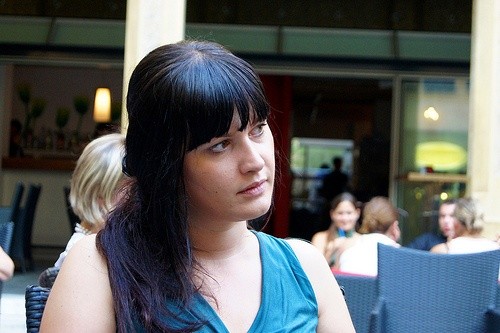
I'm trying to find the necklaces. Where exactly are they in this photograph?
[74,222,94,235]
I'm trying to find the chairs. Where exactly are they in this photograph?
[333,242,500,333]
[1,182,42,298]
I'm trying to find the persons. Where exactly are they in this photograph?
[337,196,402,277]
[38,38,357,333]
[310,192,361,268]
[50,134,136,269]
[0,244,15,282]
[427,196,500,255]
[405,198,460,252]
[311,157,352,229]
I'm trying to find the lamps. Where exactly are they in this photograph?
[93,88,111,123]
